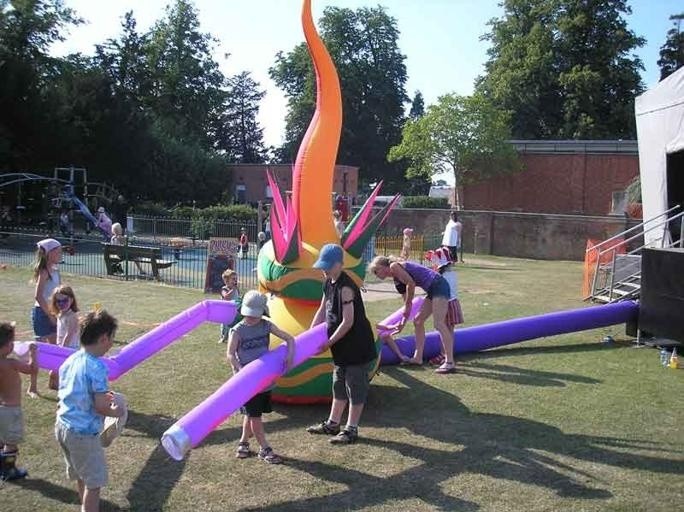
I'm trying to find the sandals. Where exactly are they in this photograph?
[400,353,456,374]
[258,447,283,463]
[235,442,249,458]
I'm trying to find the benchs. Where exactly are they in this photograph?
[104,243,178,277]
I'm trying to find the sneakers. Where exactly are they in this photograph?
[306,422,358,444]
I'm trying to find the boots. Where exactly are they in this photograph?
[0,447,28,481]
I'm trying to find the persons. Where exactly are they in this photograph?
[258,206,271,249]
[96,207,112,235]
[234,175,247,203]
[54,310,124,512]
[220,268,241,342]
[335,194,357,222]
[399,228,413,262]
[441,211,462,265]
[60,210,68,224]
[368,246,464,373]
[48,285,80,390]
[264,181,272,198]
[0,204,17,229]
[0,321,27,481]
[305,243,379,443]
[26,238,62,399]
[111,222,146,274]
[240,227,249,259]
[227,290,294,463]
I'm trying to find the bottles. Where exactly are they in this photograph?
[660,345,668,364]
[670,348,680,369]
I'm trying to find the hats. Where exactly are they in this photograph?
[240,290,269,317]
[98,206,104,212]
[425,246,453,270]
[100,391,129,447]
[312,244,343,269]
